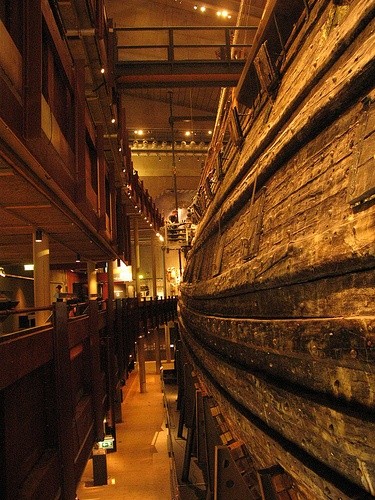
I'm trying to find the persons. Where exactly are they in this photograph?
[53,285,63,302]
[169,210,178,242]
